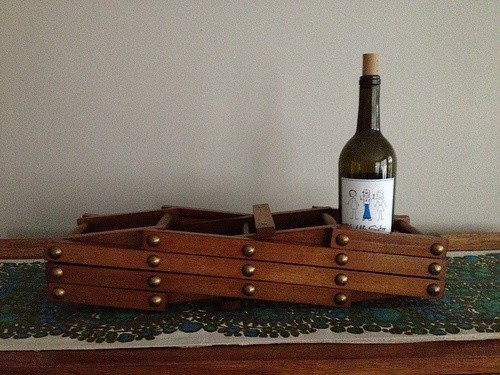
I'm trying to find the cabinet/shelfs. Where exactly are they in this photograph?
[45,203,449,311]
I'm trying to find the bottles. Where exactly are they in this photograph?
[339,52,398,234]
